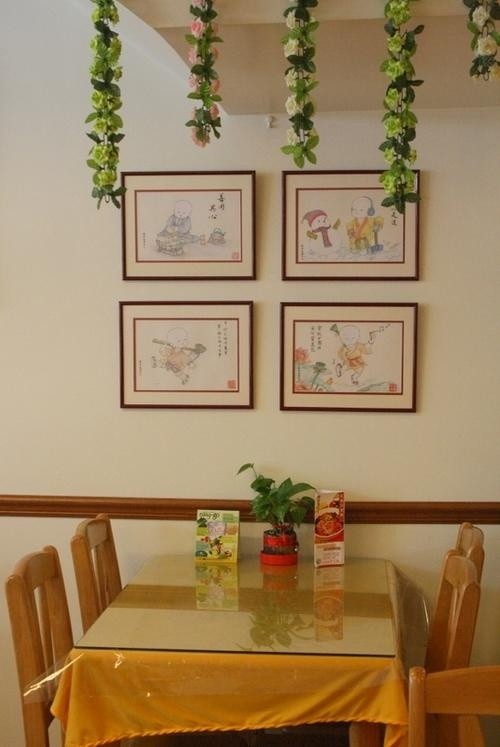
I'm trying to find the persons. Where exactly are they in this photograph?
[166,327,196,386]
[346,197,384,257]
[335,325,375,385]
[156,200,200,244]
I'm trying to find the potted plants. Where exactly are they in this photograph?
[236,462,318,565]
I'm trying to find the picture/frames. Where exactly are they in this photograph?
[282,167,420,282]
[119,299,254,410]
[120,171,258,281]
[279,301,418,412]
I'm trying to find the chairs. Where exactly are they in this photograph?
[455,523,484,583]
[424,549,482,672]
[6,545,75,745]
[407,665,500,746]
[70,513,123,634]
